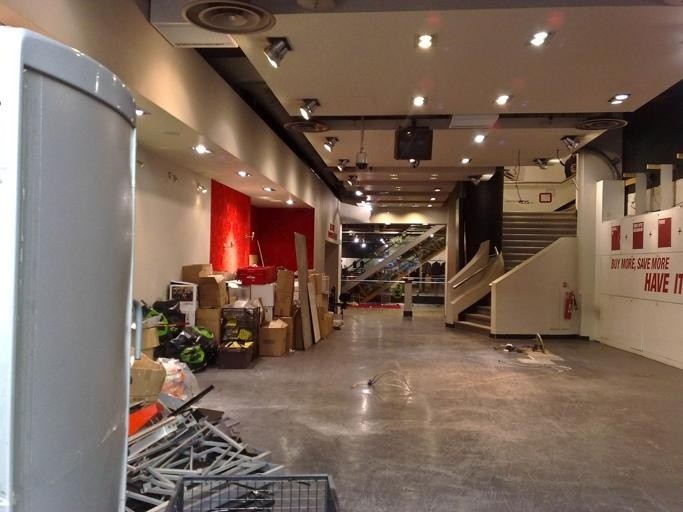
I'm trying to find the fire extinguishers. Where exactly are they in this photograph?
[565,290,579,320]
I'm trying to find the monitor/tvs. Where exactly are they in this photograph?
[395,129,433,160]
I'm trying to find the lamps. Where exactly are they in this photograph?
[262,37,357,188]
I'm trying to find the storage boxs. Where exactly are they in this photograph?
[165,264,335,367]
